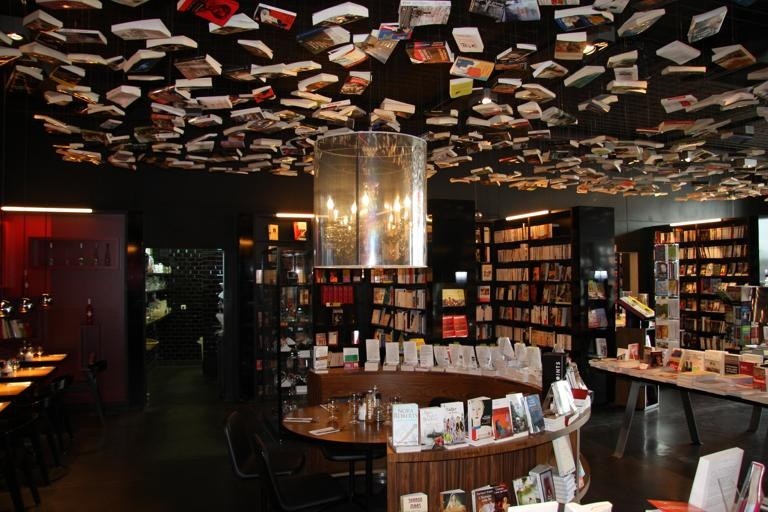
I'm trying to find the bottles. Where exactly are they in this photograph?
[348,383,400,426]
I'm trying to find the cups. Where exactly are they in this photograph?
[326,399,341,431]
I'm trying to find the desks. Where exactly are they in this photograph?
[281,398,406,512]
[1,352,67,511]
[586,352,766,459]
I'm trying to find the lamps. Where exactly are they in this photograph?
[311,131,431,273]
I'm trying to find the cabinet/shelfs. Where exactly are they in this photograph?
[145,272,173,326]
[252,202,767,440]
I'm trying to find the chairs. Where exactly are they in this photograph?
[222,410,346,512]
[321,393,387,497]
[58,357,108,440]
